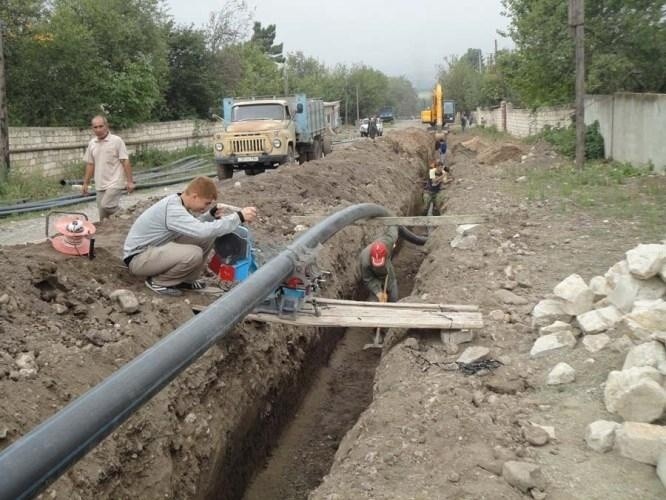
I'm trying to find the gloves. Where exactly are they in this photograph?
[376,292,389,302]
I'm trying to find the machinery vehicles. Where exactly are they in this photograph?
[419,83,464,144]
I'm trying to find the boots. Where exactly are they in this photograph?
[423,208,428,216]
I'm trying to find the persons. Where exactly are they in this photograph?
[438,137,447,164]
[121,175,258,296]
[353,237,399,337]
[81,114,132,222]
[461,112,467,133]
[420,159,453,213]
[441,166,450,181]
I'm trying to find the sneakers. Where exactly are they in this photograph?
[145,277,184,297]
[168,279,204,289]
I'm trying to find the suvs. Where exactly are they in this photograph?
[358,117,383,137]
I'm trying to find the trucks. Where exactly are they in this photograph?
[378,105,394,122]
[211,93,334,181]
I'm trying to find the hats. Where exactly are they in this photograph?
[371,240,388,268]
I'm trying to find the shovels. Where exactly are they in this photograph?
[362,265,389,351]
